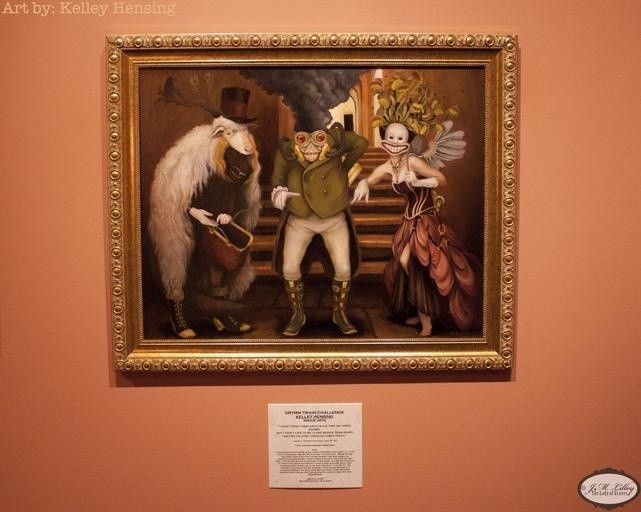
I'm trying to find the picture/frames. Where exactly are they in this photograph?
[105,32,516,375]
